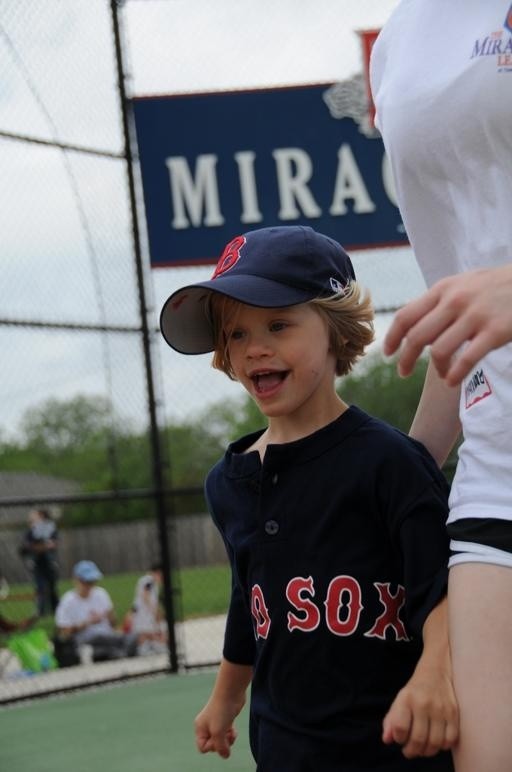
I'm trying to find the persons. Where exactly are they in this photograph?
[0,560,169,687]
[161,225,460,772]
[365,0,512,772]
[34,509,62,569]
[18,507,64,617]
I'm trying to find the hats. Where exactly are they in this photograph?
[160,224,356,355]
[73,560,104,582]
